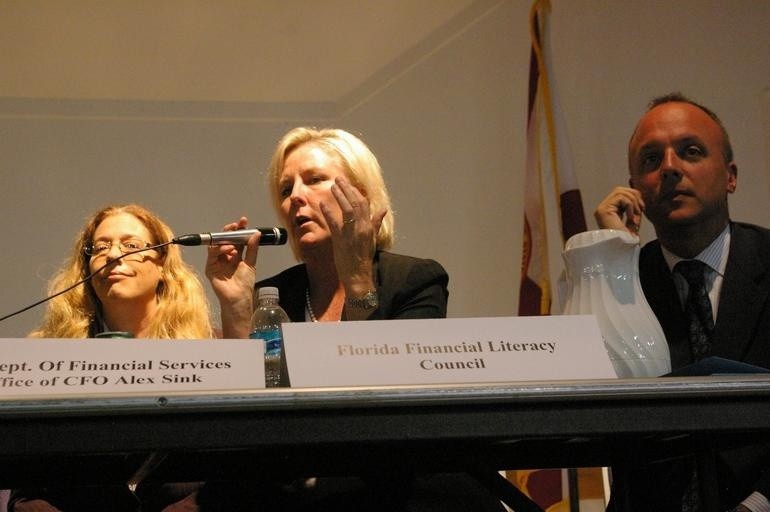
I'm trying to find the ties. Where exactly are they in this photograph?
[677,261,717,360]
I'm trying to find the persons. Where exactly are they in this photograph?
[204,125,506,511]
[593,91,770,511]
[6,203,202,512]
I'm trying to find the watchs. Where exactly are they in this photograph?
[339,292,383,312]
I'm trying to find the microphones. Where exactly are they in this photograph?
[172,225,290,250]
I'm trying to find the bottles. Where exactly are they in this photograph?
[247,285,292,390]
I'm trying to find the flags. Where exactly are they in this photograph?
[515,14,588,315]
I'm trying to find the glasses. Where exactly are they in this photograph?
[82,234,159,259]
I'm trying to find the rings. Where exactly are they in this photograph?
[342,216,357,223]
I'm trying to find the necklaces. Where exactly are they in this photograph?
[306,286,344,323]
